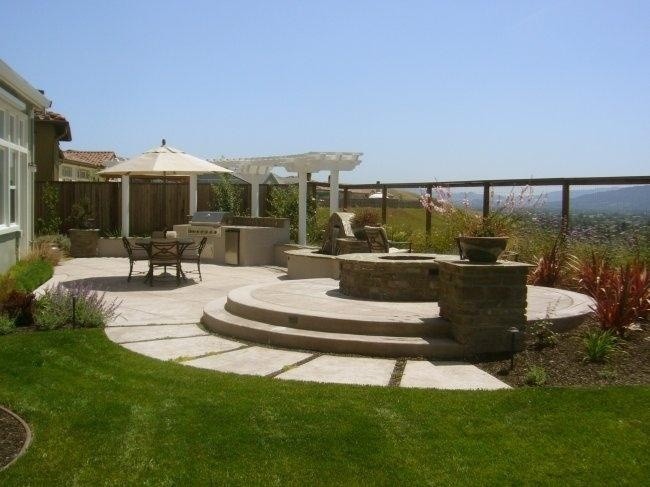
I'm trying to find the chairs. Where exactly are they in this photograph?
[122,235,207,287]
[364,225,412,252]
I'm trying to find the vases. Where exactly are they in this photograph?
[458,237,508,264]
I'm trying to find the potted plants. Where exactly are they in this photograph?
[66,194,102,257]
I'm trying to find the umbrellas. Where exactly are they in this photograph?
[95,137,234,229]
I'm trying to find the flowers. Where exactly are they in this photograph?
[419,176,547,237]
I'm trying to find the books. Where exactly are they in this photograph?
[166,224,191,239]
